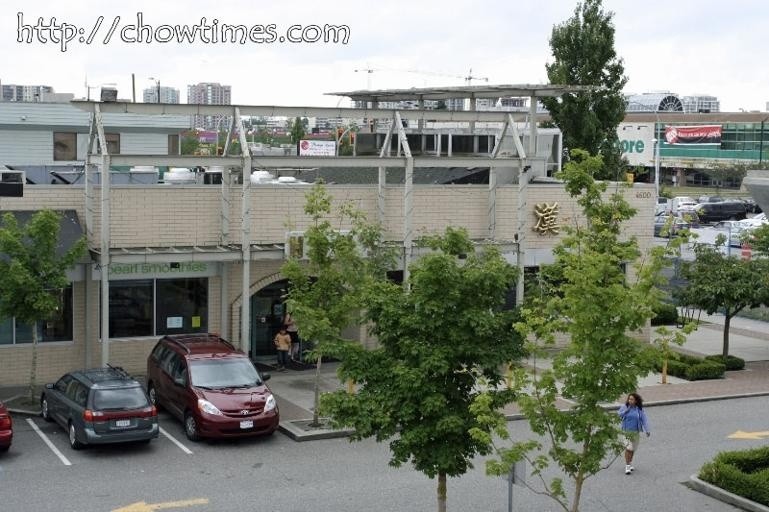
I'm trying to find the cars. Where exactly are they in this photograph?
[654,194,769,247]
[42,367,159,450]
[0,400,13,451]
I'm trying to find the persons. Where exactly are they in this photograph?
[617,392,651,474]
[285,312,301,362]
[272,328,291,372]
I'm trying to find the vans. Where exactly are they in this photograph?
[148,334,279,442]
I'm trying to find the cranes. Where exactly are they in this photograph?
[354,65,488,90]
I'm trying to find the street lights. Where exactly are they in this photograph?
[148,78,160,103]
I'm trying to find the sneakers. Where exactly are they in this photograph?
[624,462,635,474]
